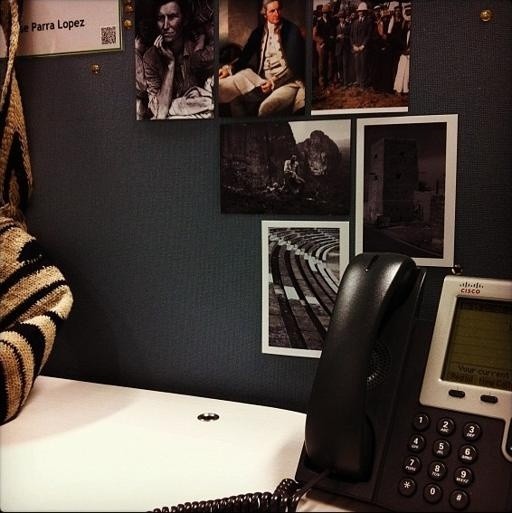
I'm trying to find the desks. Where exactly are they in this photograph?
[1,374,308,513]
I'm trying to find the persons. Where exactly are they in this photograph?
[284,154,300,195]
[142,1,215,121]
[312,0,411,96]
[218,0,305,117]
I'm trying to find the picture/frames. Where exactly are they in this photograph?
[354,114,458,271]
[260,221,349,358]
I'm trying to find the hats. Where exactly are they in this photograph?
[357,3,367,11]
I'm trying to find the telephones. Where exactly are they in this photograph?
[294,253,512,512]
